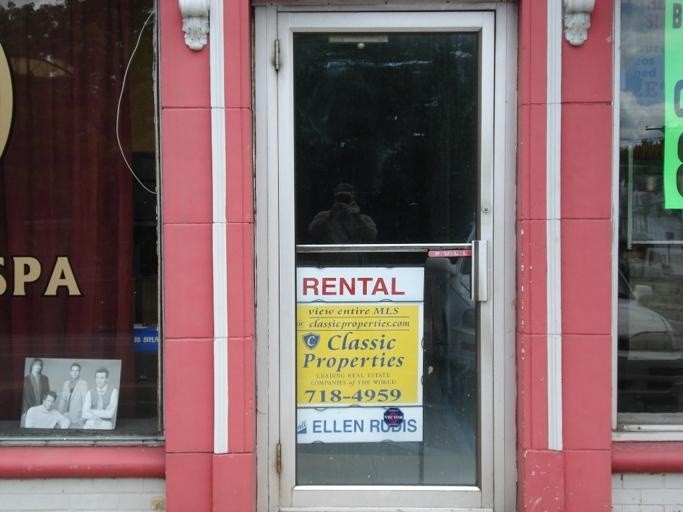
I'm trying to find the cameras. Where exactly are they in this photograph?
[333,191,352,205]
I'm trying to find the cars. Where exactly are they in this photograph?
[425,221,680,413]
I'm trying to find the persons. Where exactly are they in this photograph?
[20,359,117,430]
[307,181,378,267]
